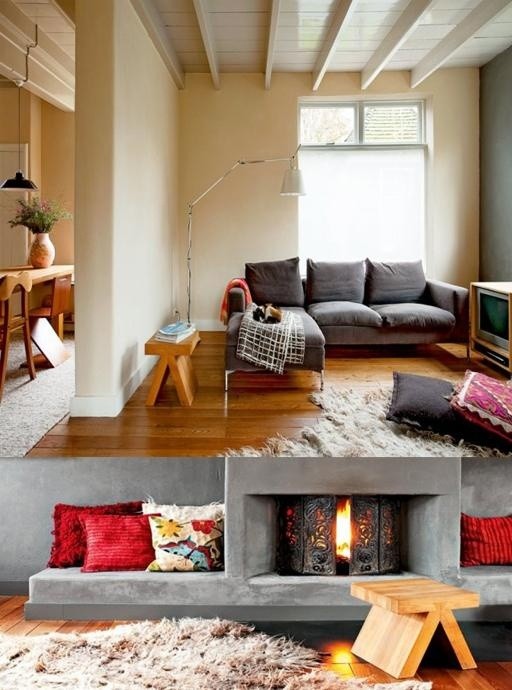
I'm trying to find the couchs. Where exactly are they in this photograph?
[224,274,470,390]
[30,458,511,626]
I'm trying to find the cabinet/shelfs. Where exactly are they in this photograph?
[469,281,512,375]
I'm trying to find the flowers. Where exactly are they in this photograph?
[6,195,72,232]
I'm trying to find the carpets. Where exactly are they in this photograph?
[217,379,512,457]
[0,327,77,457]
[1,616,434,690]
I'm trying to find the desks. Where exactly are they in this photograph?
[0,264,74,295]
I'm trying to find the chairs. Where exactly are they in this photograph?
[0,273,38,406]
[20,274,73,369]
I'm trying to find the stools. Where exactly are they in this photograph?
[145,330,201,407]
[351,578,480,679]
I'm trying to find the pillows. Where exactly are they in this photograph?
[48,501,151,568]
[145,516,225,572]
[141,502,225,522]
[79,513,160,573]
[461,512,512,568]
[451,369,512,442]
[387,372,457,437]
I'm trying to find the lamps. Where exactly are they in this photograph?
[0,78,39,191]
[185,144,306,325]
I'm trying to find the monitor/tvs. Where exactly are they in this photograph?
[476,289,510,348]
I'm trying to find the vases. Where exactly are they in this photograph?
[30,233,55,268]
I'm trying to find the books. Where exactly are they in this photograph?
[155,322,196,344]
